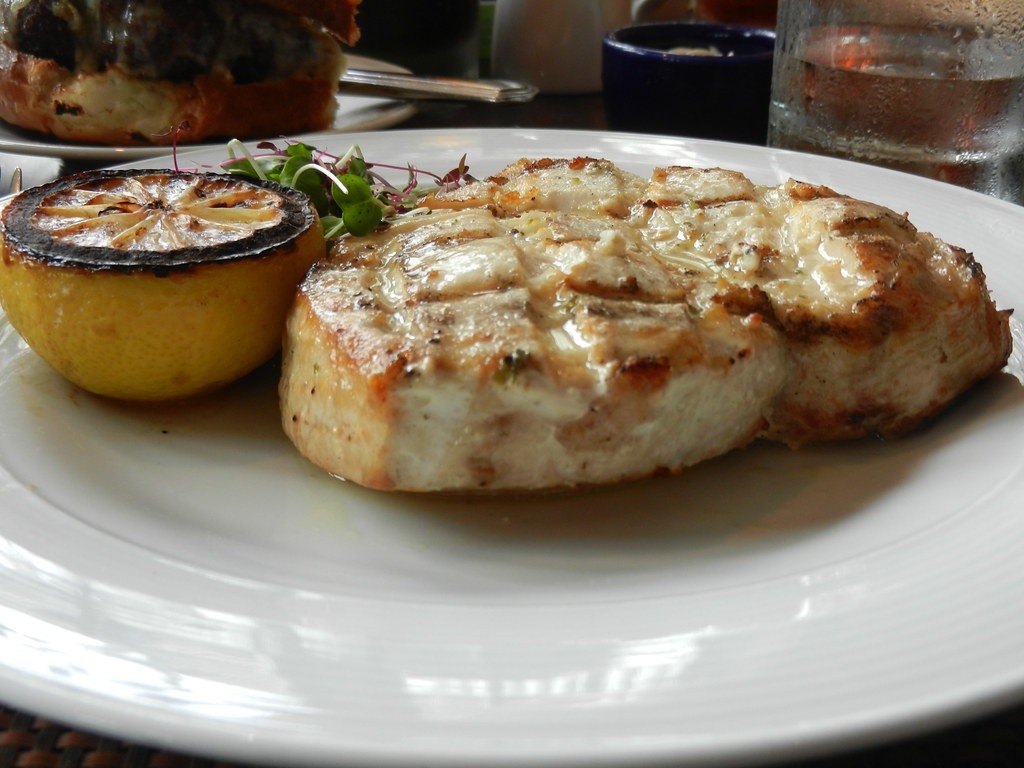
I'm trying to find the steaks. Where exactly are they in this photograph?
[280,150,1013,496]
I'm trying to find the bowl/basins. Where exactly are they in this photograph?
[603,23,776,160]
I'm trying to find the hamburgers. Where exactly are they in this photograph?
[0,0,358,140]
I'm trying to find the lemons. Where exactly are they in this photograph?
[1,168,321,401]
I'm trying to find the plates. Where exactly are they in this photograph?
[0,50,422,164]
[0,126,1024,766]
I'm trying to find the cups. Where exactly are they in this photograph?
[765,2,1022,219]
